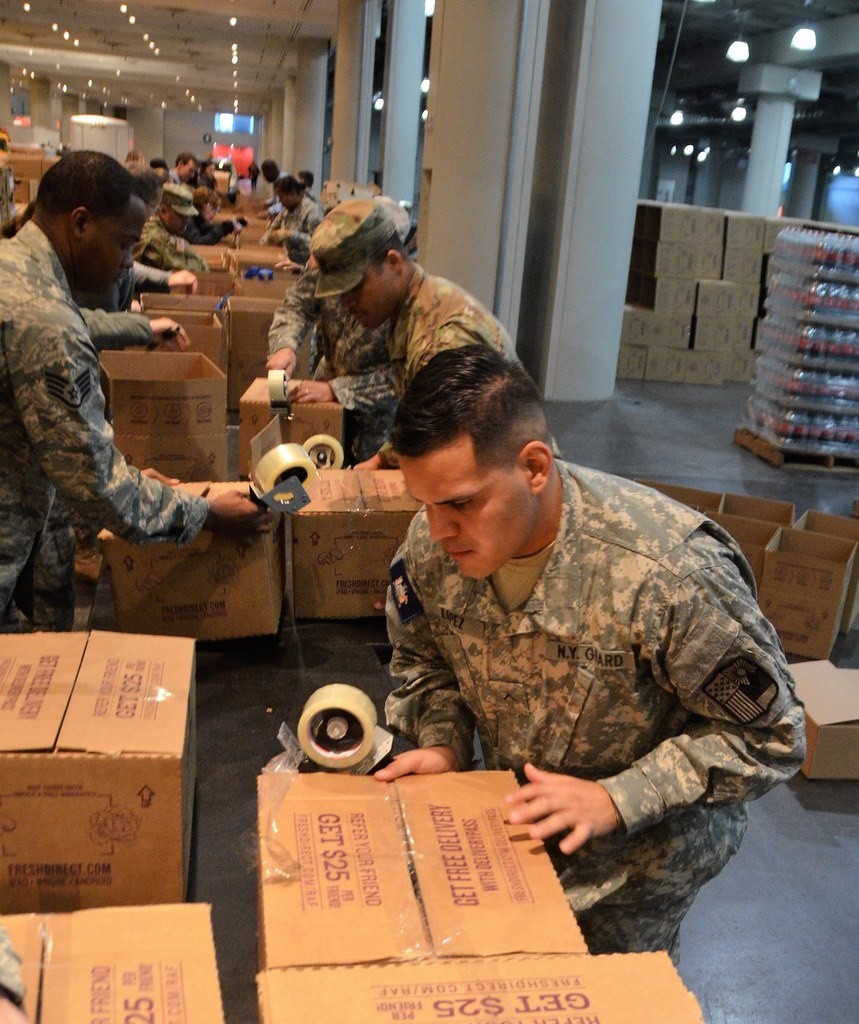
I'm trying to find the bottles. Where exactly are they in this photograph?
[742,226,859,455]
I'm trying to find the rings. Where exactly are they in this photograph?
[305,387,310,394]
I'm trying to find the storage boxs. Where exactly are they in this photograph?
[322,180,384,209]
[787,659,859,782]
[0,631,196,915]
[0,900,227,1024]
[169,171,300,298]
[7,144,63,231]
[239,376,344,477]
[97,481,285,641]
[139,292,311,409]
[287,468,425,620]
[255,767,588,970]
[634,477,859,662]
[254,950,706,1024]
[617,200,859,386]
[98,349,229,484]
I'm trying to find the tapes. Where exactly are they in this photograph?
[255,442,316,500]
[297,684,378,769]
[265,368,289,399]
[303,433,345,472]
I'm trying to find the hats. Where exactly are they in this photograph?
[161,184,200,216]
[372,195,412,244]
[310,199,395,298]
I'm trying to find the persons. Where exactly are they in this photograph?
[0,149,418,314]
[0,150,274,635]
[265,195,563,471]
[374,343,806,966]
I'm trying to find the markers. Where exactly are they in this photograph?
[145,325,178,352]
